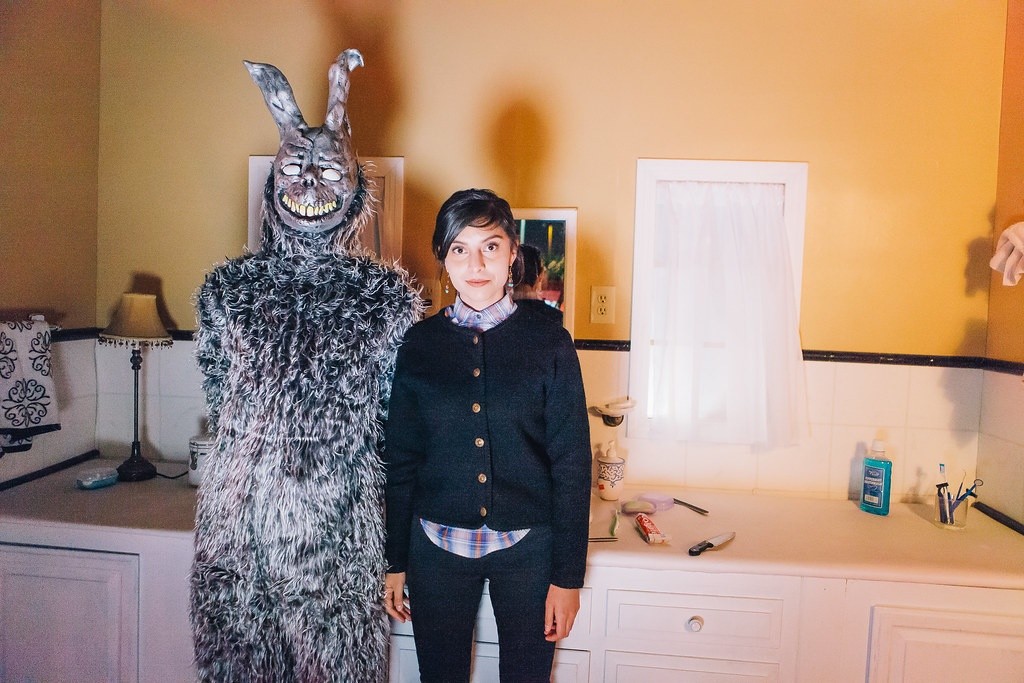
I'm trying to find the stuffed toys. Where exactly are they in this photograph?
[189,45,432,682]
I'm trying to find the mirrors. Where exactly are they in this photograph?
[508,207,578,344]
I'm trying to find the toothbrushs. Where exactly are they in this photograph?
[938,462,953,525]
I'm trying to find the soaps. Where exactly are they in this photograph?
[621,500,656,514]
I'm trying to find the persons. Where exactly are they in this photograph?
[510,244,564,327]
[379,182,592,683]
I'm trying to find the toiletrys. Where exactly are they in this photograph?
[859,438,893,516]
[633,512,673,545]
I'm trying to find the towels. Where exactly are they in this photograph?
[0,319,63,460]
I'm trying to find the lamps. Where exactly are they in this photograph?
[97,291,175,476]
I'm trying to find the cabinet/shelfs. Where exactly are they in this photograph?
[0,456,1023,682]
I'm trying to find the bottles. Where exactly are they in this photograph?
[858,439,894,518]
[186,435,217,489]
